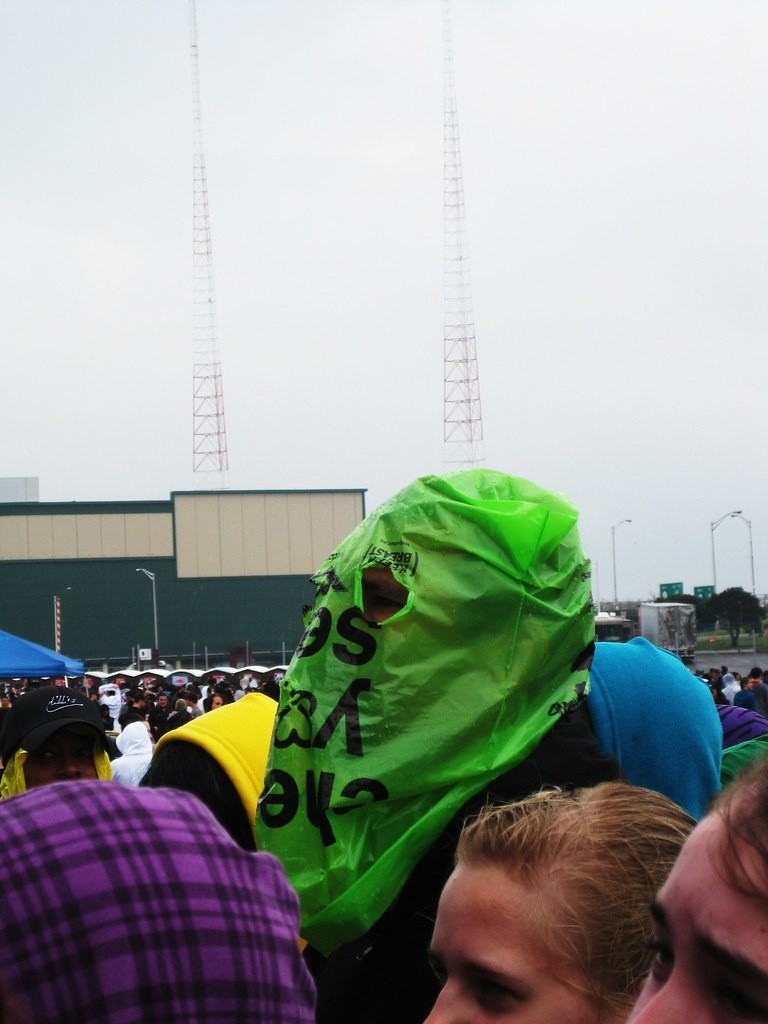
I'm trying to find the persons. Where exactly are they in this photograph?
[1,466,767,1023]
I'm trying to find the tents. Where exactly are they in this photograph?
[0,629,92,698]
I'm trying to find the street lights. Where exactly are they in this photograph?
[731,514,756,597]
[54,587,72,652]
[711,510,742,593]
[136,568,157,649]
[613,518,632,604]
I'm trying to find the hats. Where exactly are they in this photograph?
[3,685,111,765]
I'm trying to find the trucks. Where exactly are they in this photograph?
[638,601,698,664]
